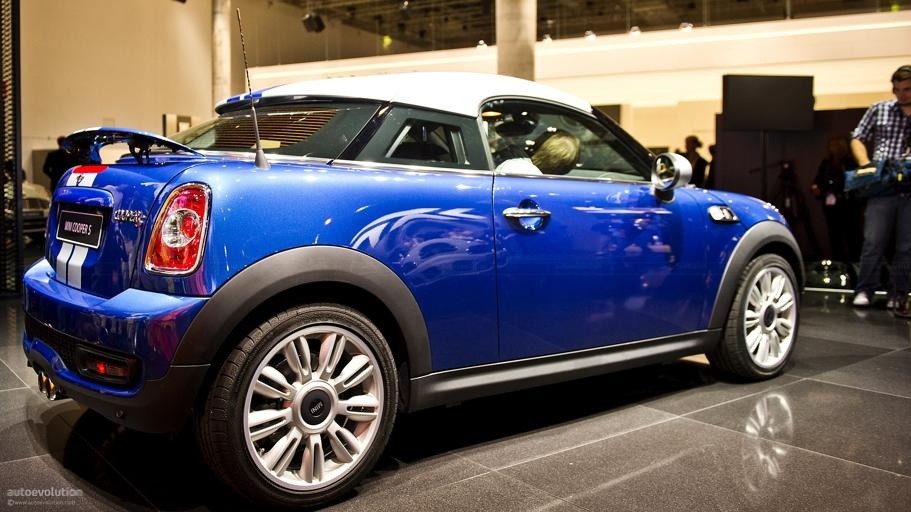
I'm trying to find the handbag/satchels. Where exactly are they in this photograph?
[844,157,910,200]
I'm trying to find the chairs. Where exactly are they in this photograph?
[397,141,447,161]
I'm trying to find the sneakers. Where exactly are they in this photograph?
[887,297,908,308]
[853,292,870,306]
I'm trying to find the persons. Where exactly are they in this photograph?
[494,128,582,176]
[42,136,72,195]
[810,137,858,262]
[679,135,707,185]
[848,65,911,309]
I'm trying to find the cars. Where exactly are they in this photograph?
[2,163,51,256]
[18,74,803,502]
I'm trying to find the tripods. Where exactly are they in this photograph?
[748,161,821,260]
